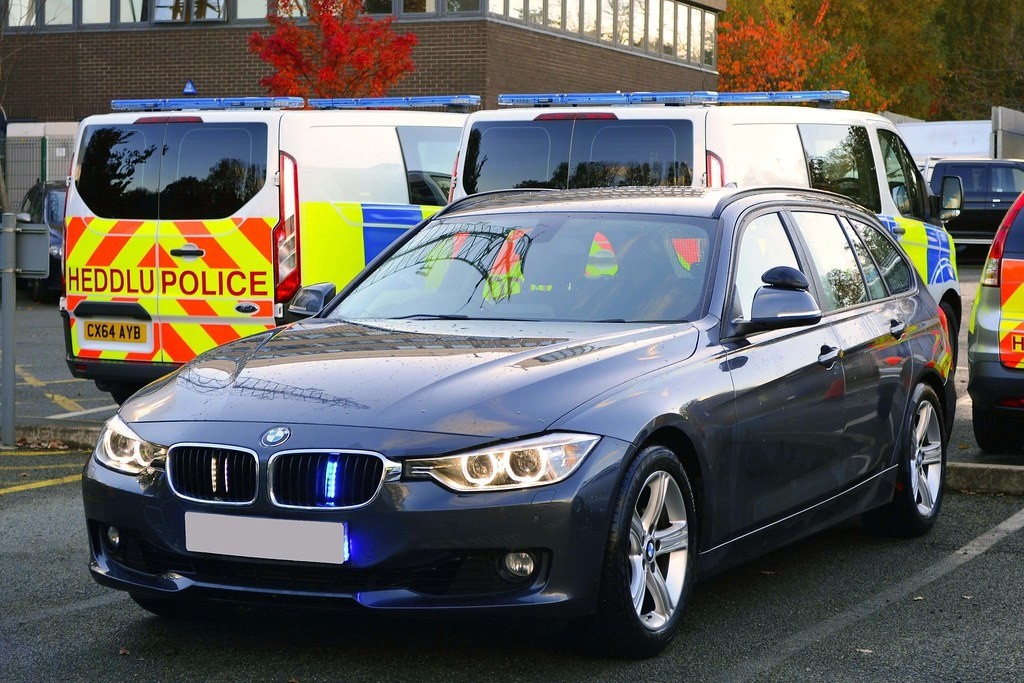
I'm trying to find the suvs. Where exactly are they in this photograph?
[14,177,68,297]
[900,156,1023,263]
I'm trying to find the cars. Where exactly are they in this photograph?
[965,186,1024,457]
[81,183,958,654]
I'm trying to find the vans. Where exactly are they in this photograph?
[60,86,965,401]
